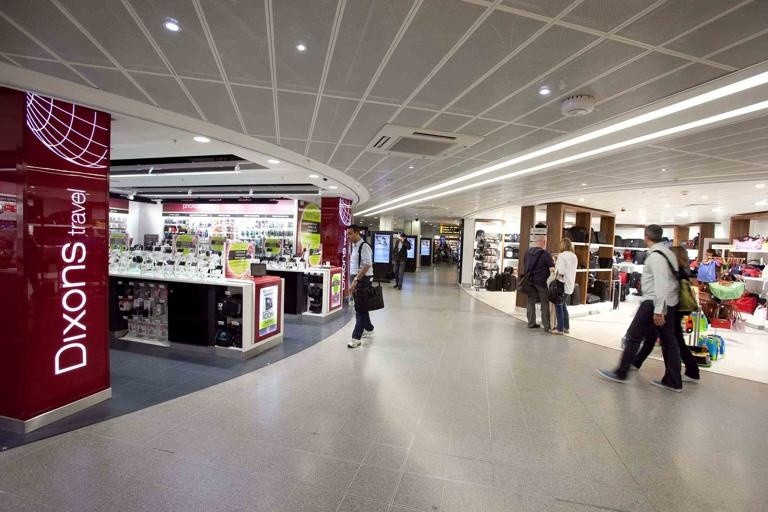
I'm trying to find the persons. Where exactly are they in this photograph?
[347,224,384,348]
[517,235,555,332]
[394,233,411,290]
[547,238,579,334]
[595,225,684,392]
[704,248,722,259]
[630,247,703,383]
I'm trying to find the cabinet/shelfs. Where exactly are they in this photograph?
[164,217,187,245]
[108,267,284,362]
[435,239,458,263]
[729,211,768,333]
[210,217,238,245]
[473,230,502,286]
[615,224,674,267]
[187,217,210,245]
[266,266,343,318]
[502,233,520,280]
[262,217,295,256]
[561,204,615,304]
[238,217,262,256]
[108,209,128,242]
[676,222,714,278]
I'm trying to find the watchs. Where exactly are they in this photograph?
[354,276,359,280]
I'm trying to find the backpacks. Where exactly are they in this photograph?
[353,242,384,312]
[548,270,565,306]
[517,249,547,294]
[504,245,514,258]
[652,250,699,313]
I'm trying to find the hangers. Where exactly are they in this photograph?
[698,257,745,324]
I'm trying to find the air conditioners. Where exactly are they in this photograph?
[365,124,486,161]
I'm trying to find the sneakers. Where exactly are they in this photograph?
[594,365,631,386]
[651,377,684,393]
[362,329,374,337]
[563,330,570,335]
[346,338,362,348]
[549,329,565,336]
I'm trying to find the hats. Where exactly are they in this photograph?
[400,231,406,237]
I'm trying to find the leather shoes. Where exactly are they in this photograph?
[393,283,397,289]
[528,324,542,328]
[398,287,402,290]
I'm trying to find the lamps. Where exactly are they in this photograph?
[128,191,136,199]
[235,163,240,171]
[560,95,595,117]
[682,191,689,195]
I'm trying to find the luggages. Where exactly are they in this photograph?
[503,273,516,292]
[485,273,496,290]
[495,271,504,292]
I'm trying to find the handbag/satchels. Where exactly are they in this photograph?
[598,257,614,268]
[732,231,768,251]
[697,262,716,284]
